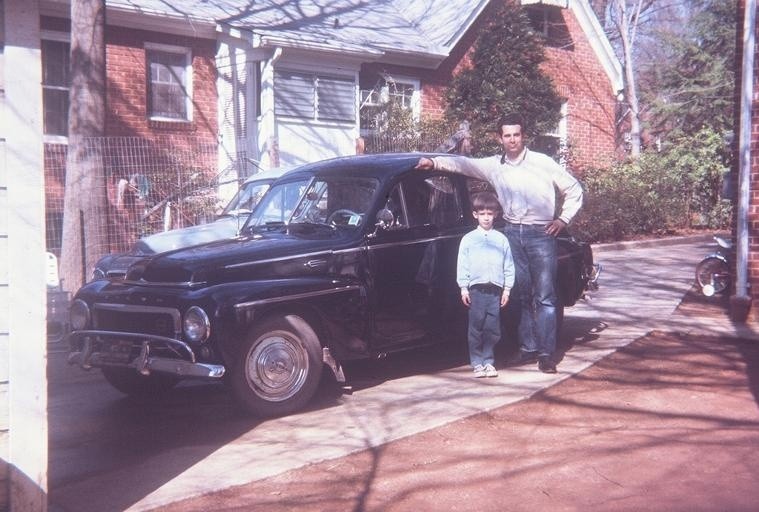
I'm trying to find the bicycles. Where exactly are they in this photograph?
[692,230,743,295]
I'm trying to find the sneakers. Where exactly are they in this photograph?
[485,363,498,377]
[473,365,486,378]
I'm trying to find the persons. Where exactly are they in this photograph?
[455,193,516,379]
[416,114,585,373]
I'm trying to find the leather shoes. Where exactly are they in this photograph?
[538,357,556,373]
[502,350,537,367]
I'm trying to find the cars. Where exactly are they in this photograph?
[66,150,604,431]
[85,159,479,290]
